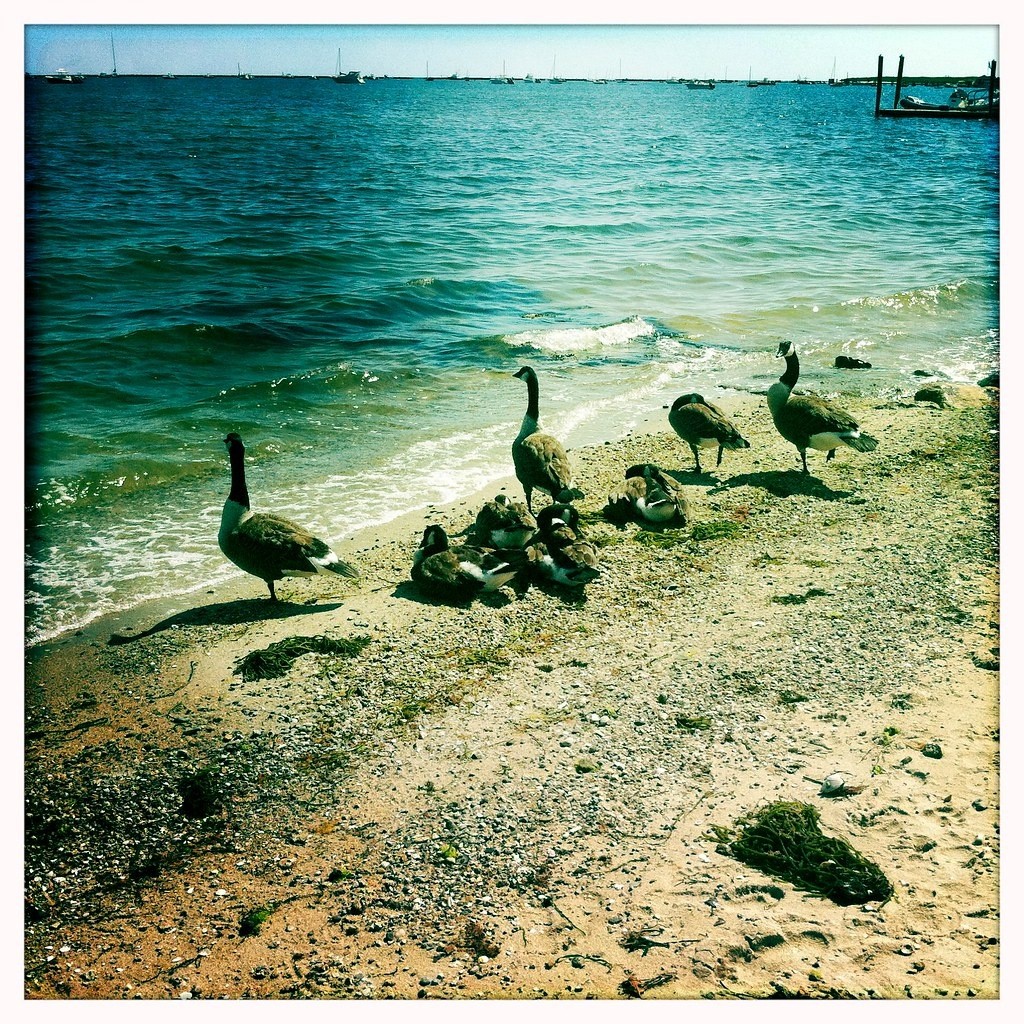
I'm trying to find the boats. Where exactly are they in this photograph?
[240,73,256,80]
[163,72,176,79]
[97,69,119,78]
[796,77,826,84]
[666,74,715,90]
[746,66,781,87]
[490,54,566,84]
[899,88,1000,110]
[46,68,83,84]
[593,79,608,84]
[332,48,365,84]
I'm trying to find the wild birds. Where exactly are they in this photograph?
[609,461,694,527]
[511,366,584,511]
[518,505,601,585]
[668,393,750,471]
[411,525,530,594]
[767,340,880,477]
[476,495,536,545]
[219,433,360,603]
